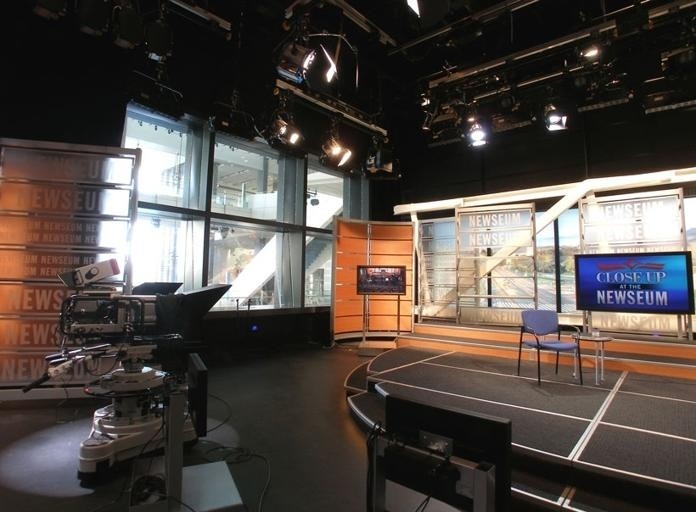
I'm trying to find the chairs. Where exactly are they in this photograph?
[516,308,587,389]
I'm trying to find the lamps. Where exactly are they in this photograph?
[534,100,568,133]
[305,42,342,92]
[316,135,354,169]
[460,113,489,148]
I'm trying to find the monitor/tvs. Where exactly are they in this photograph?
[574,251,695,314]
[357,264,406,294]
[188,353,208,437]
[386,395,511,512]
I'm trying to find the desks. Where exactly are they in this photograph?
[569,337,614,386]
